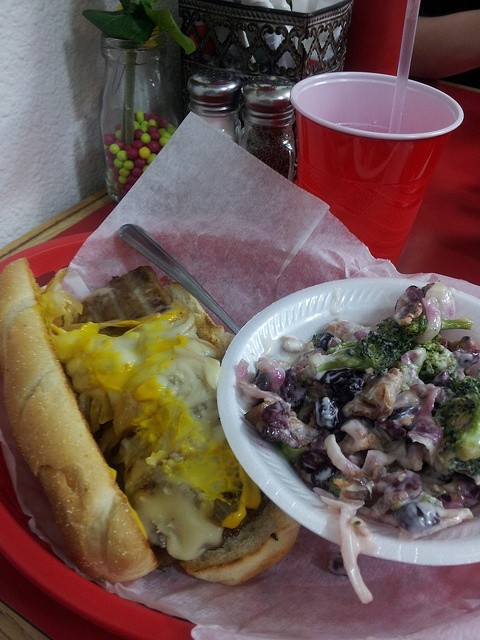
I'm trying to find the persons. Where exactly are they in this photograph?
[409,0,480,89]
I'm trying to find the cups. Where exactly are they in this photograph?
[282,69,466,265]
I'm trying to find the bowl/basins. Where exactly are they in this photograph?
[0,231,480,639]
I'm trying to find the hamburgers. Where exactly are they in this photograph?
[0,257,302,587]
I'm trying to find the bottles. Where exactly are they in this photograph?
[182,66,241,145]
[239,74,295,180]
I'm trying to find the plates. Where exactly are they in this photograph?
[216,272,480,569]
